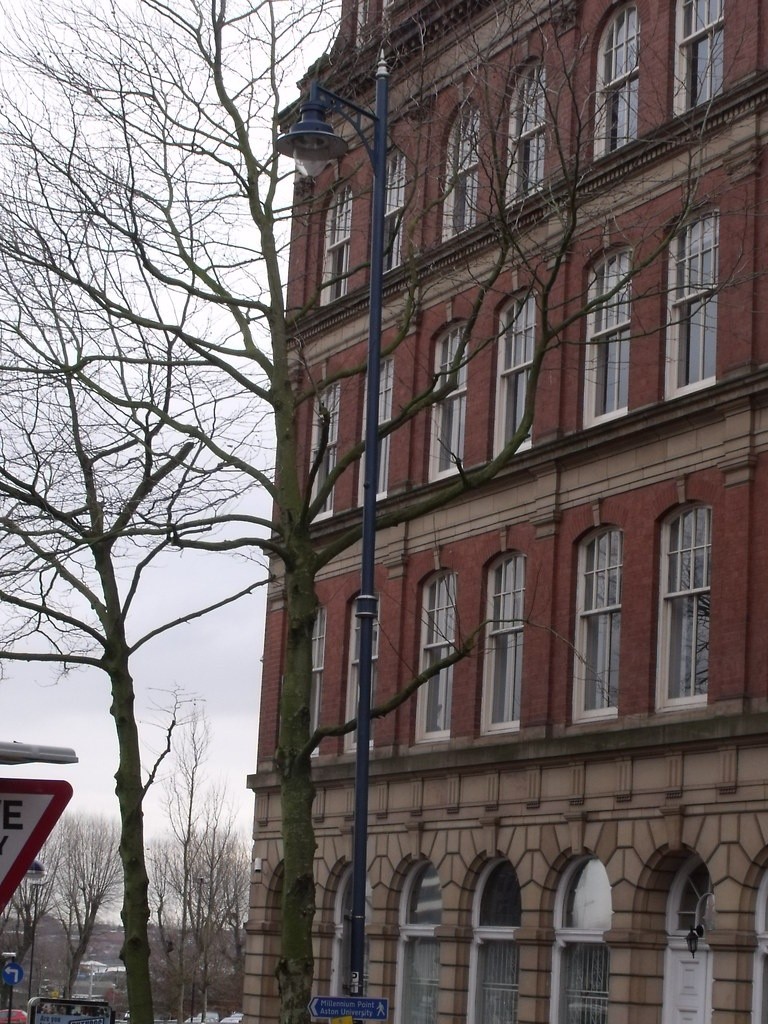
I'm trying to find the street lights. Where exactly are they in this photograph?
[275,49,390,997]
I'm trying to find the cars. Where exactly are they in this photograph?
[0,1010,27,1024]
[220,1014,244,1023]
[185,1013,219,1023]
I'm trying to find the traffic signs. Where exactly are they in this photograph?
[307,996,388,1022]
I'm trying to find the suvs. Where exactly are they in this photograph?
[123,1011,130,1020]
